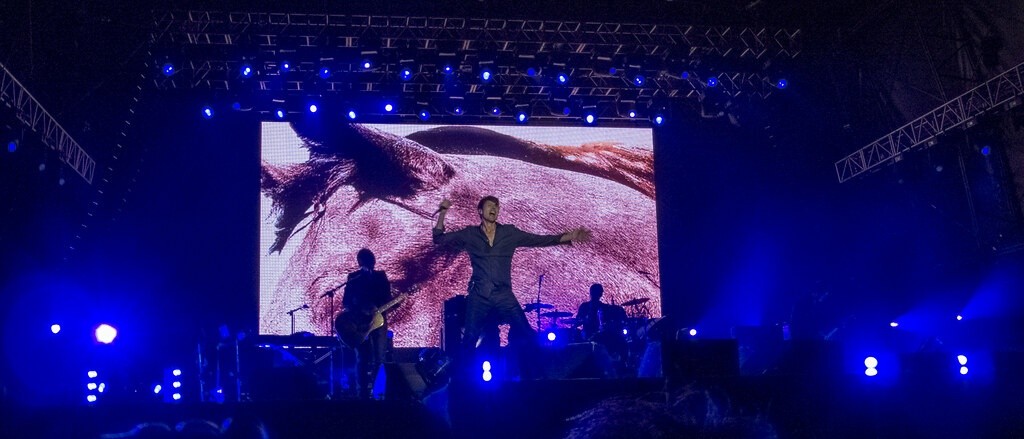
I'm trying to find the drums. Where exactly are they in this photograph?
[596,305,653,369]
[561,342,611,388]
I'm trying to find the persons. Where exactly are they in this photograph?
[577,284,609,336]
[342,249,391,399]
[433,195,594,381]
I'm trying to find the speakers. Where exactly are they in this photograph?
[444,297,500,361]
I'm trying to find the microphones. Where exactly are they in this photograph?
[431,206,446,218]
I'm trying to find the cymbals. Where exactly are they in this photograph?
[621,298,649,306]
[560,318,579,324]
[540,311,573,317]
[523,303,554,308]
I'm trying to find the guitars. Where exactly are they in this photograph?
[334,282,422,350]
